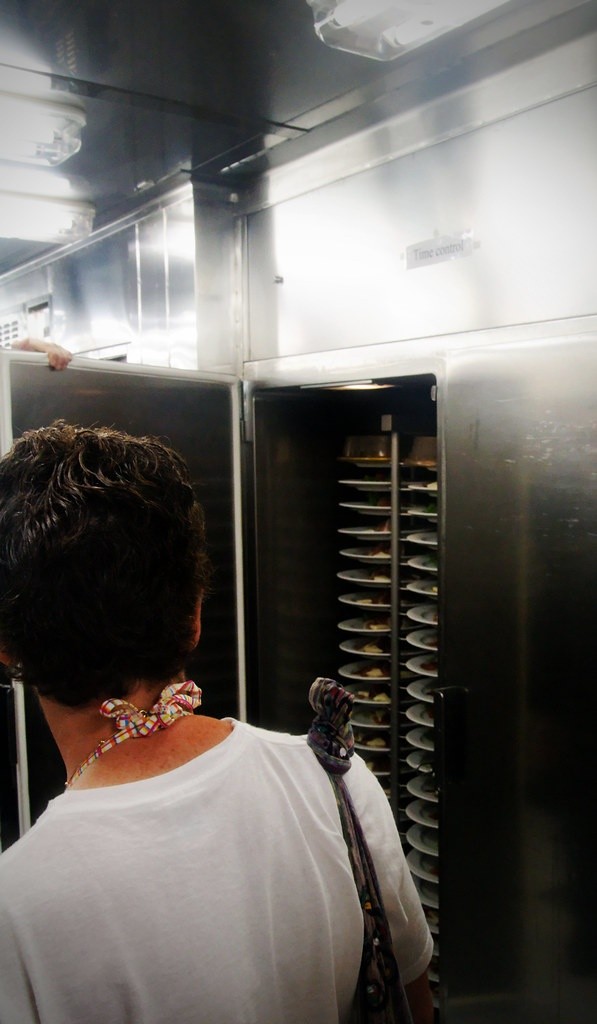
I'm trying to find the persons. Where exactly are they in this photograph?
[10,336,72,370]
[0,425,436,1024]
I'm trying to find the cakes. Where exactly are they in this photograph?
[344,434,393,458]
[407,436,437,462]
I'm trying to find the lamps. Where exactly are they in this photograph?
[0,90,98,245]
[308,0,508,63]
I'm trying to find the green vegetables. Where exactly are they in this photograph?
[364,472,439,569]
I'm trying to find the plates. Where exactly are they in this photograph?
[338,479,391,491]
[336,594,442,1010]
[340,503,391,516]
[406,532,439,545]
[408,484,440,497]
[338,455,391,466]
[339,525,432,535]
[408,557,439,576]
[337,568,411,587]
[406,581,439,599]
[339,549,419,563]
[408,506,440,525]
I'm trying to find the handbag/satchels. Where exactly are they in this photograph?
[301,677,416,1024]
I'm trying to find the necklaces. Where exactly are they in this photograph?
[64,680,203,788]
